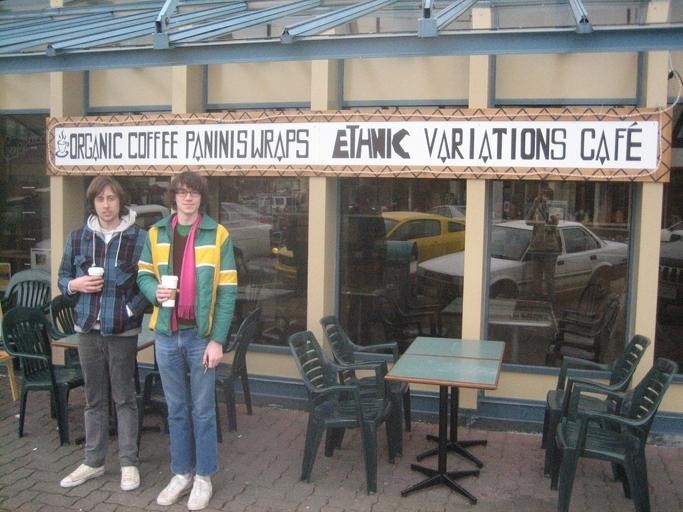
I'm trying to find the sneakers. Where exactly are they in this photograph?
[154,471,193,507]
[119,464,140,491]
[58,462,105,489]
[183,474,214,511]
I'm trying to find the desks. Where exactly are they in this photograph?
[232,284,294,345]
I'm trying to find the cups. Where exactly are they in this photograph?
[160,275,178,307]
[87,267,104,276]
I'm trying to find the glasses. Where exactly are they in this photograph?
[173,189,200,198]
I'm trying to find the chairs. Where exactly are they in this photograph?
[370,261,619,362]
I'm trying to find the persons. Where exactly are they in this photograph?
[347,185,386,345]
[526,187,562,304]
[137,172,238,511]
[286,193,308,296]
[57,176,151,491]
